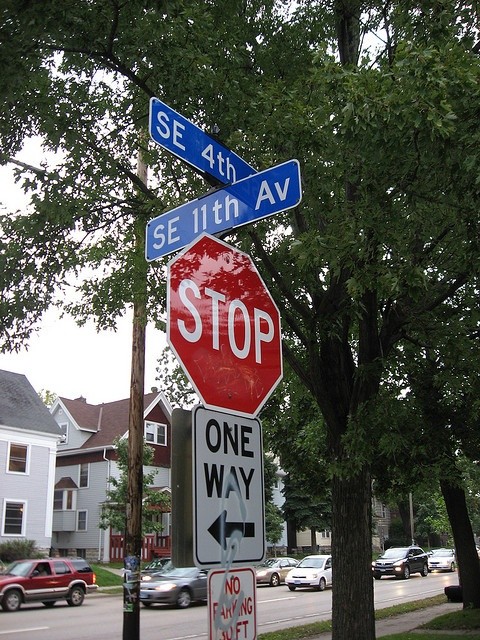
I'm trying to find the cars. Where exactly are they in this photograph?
[140,561,207,607]
[141,557,173,580]
[284,555,332,591]
[256,557,299,586]
[427,549,457,572]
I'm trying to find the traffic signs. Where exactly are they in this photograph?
[190,403,267,569]
[207,567,256,640]
[148,96,259,186]
[144,158,302,262]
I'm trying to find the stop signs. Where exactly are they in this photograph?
[166,231,283,419]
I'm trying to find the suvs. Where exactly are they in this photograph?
[0,555,99,611]
[372,545,429,579]
